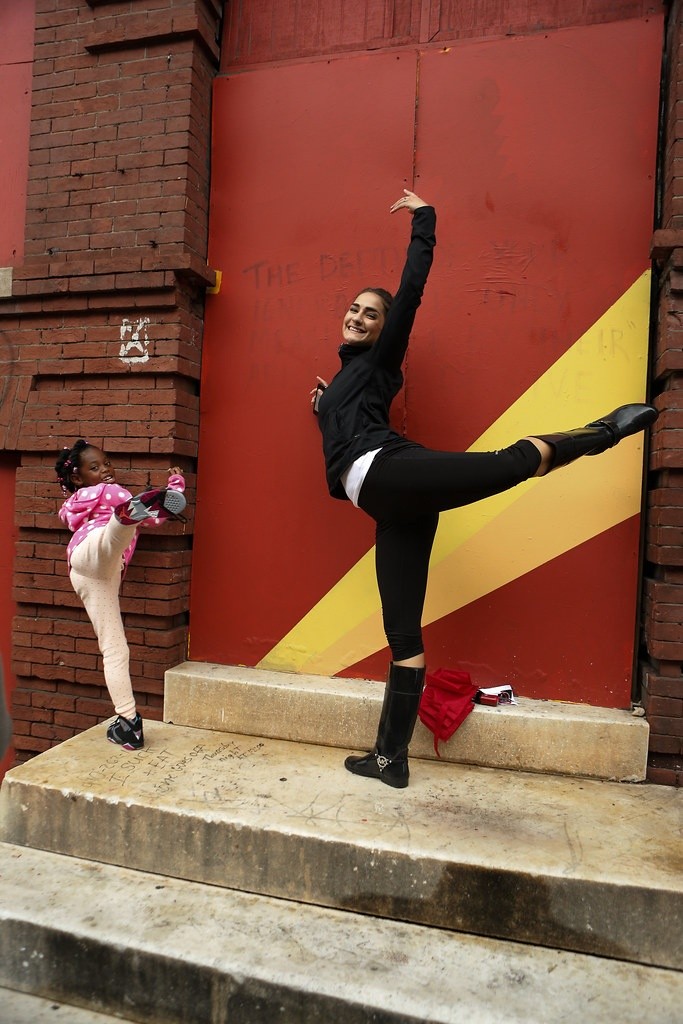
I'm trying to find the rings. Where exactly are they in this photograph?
[403,197,407,202]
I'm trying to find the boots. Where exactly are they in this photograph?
[526,403,659,477]
[344,661,427,789]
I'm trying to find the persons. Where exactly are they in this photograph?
[55,438,187,749]
[304,188,659,791]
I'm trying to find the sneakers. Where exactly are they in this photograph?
[107,711,144,750]
[114,488,186,525]
